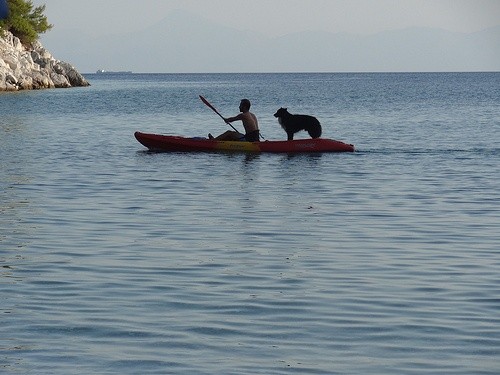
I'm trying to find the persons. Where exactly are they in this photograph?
[207,98,260,142]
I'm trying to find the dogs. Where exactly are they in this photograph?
[273,107,322,141]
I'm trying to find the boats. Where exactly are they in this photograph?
[133,130,354,154]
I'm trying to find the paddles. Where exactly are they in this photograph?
[198,94,239,133]
